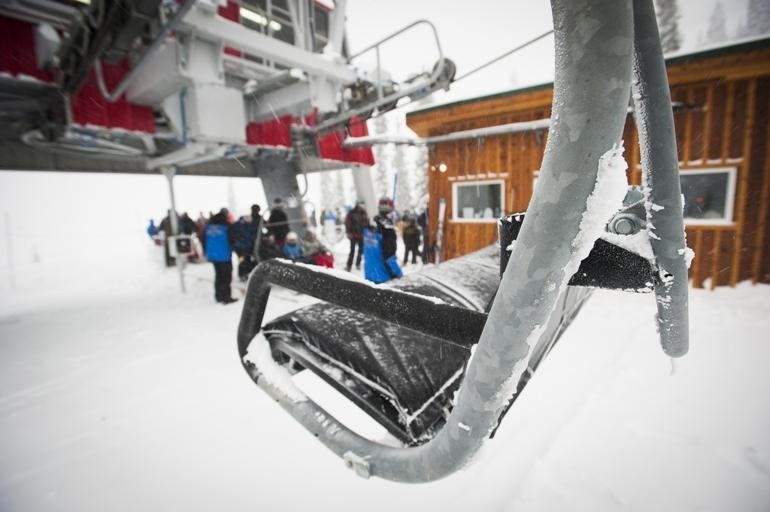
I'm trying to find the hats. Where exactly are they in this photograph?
[287,232,297,240]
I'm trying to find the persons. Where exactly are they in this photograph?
[148,195,431,284]
[202,208,239,304]
